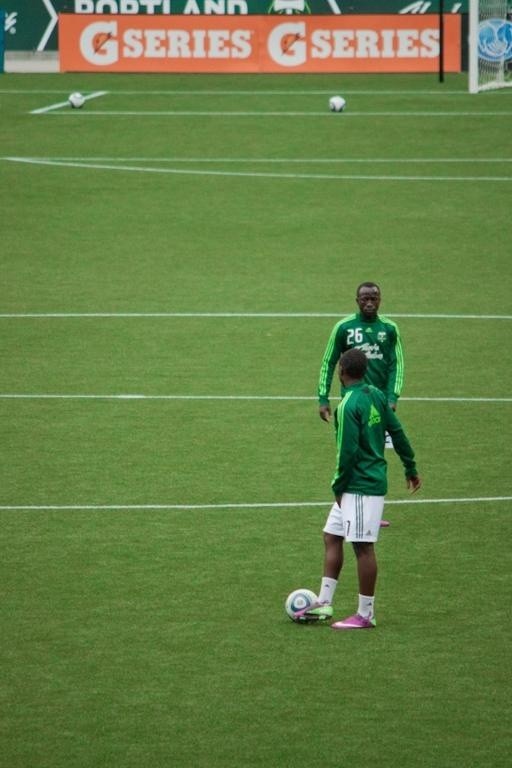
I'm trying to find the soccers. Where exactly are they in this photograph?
[285,589,318,621]
[69,92,84,109]
[329,96,346,112]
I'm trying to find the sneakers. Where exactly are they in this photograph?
[330,611,377,629]
[293,600,335,621]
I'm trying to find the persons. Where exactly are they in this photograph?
[293,348,421,630]
[317,281,404,527]
